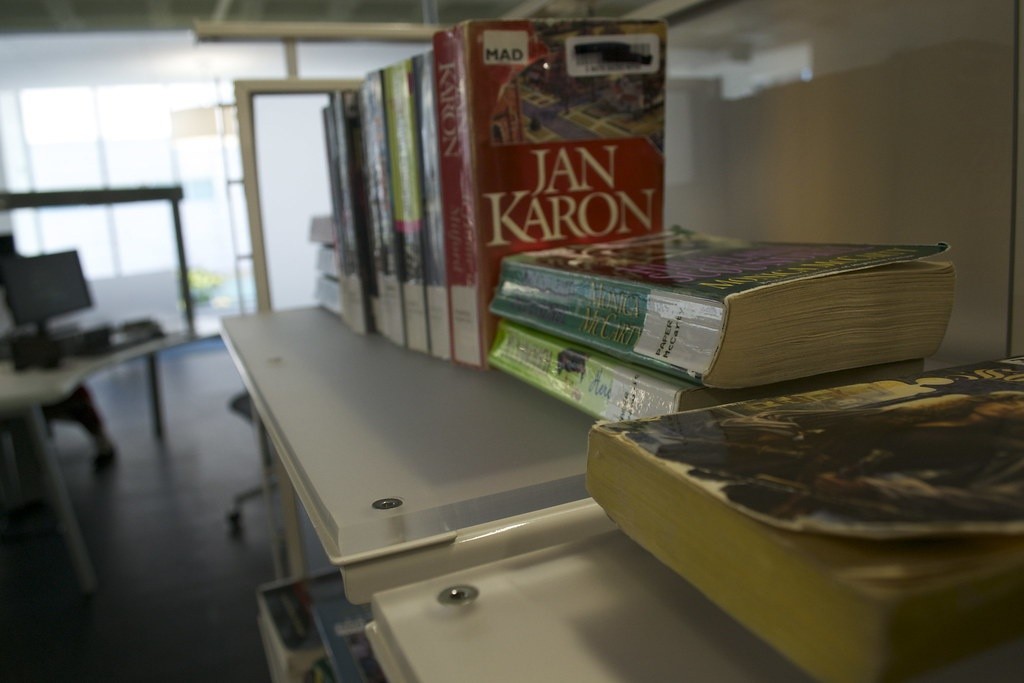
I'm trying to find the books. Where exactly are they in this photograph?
[306,18,666,368]
[484,224,956,430]
[587,355,1024,683]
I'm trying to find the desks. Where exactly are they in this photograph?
[218,303,1024,683]
[0,329,189,591]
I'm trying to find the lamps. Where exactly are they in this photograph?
[190,0,456,41]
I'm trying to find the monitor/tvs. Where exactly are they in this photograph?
[0,250,93,334]
